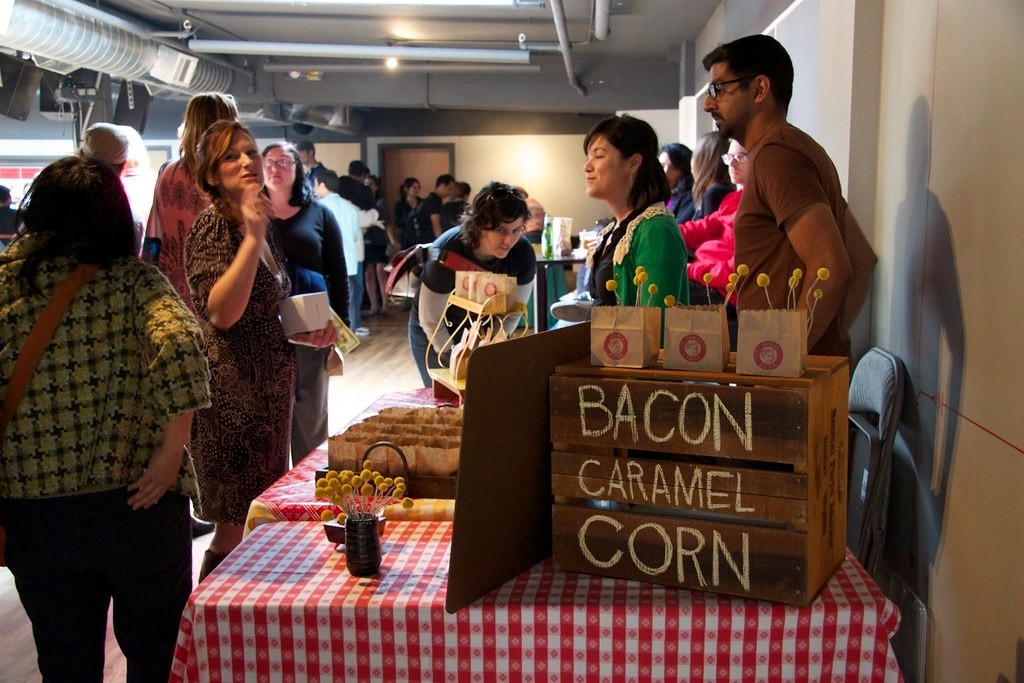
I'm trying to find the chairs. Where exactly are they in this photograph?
[849,346,905,585]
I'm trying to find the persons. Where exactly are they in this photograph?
[584,116,692,349]
[0,185,22,245]
[701,34,879,355]
[549,133,750,320]
[258,140,353,468]
[184,118,296,586]
[297,140,474,336]
[0,157,211,683]
[76,121,150,257]
[143,90,239,310]
[406,182,538,390]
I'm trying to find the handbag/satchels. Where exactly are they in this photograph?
[384,243,486,299]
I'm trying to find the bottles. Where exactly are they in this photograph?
[540,214,551,256]
[578,231,597,249]
[544,223,554,260]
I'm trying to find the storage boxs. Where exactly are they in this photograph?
[280,291,331,337]
[546,352,852,609]
[323,516,386,544]
[316,465,457,499]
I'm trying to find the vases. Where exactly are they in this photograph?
[344,515,383,577]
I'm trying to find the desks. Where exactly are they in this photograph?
[242,387,464,522]
[534,248,587,334]
[167,521,905,683]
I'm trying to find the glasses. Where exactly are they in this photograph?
[721,153,748,165]
[706,74,758,98]
[264,158,298,167]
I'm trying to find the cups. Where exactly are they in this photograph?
[574,249,588,260]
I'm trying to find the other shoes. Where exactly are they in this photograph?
[550,293,594,321]
[354,327,371,336]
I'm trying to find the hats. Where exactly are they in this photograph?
[296,140,313,150]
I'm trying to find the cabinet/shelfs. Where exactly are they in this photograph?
[424,288,530,409]
